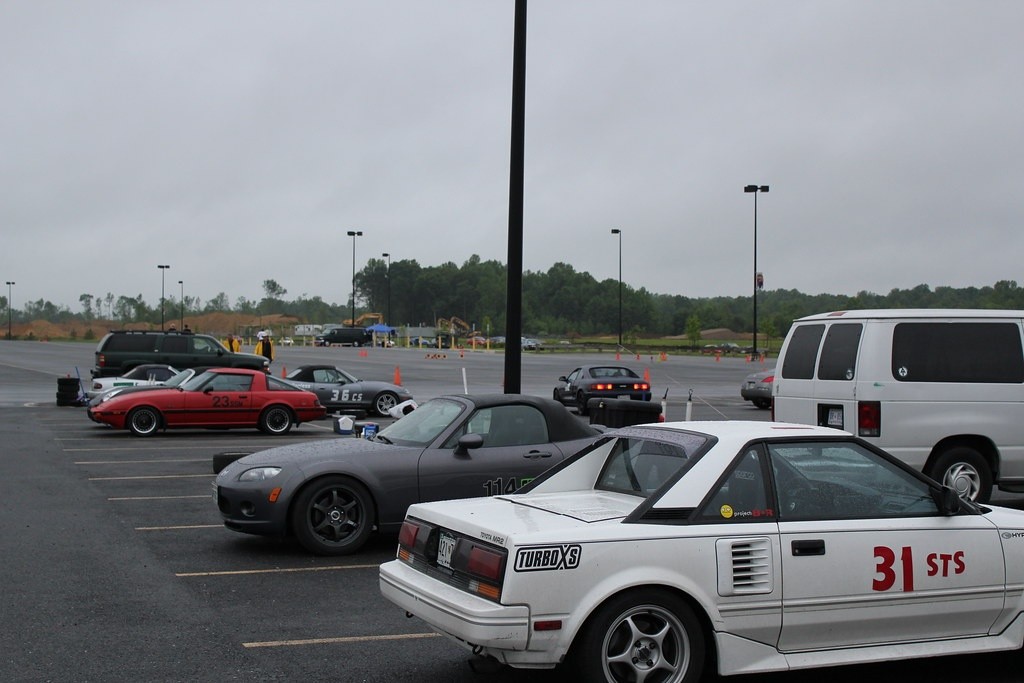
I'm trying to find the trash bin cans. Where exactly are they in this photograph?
[355,422,379,439]
[586,398,662,429]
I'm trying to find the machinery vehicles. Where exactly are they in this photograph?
[438,316,482,338]
[343,313,383,327]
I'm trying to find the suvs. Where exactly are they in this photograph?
[90,329,272,381]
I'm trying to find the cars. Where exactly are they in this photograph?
[553,365,652,417]
[521,337,545,351]
[378,339,395,347]
[410,336,429,346]
[210,394,617,557]
[559,341,571,344]
[741,368,776,409]
[85,364,414,438]
[279,337,294,345]
[468,336,505,346]
[699,343,764,354]
[378,420,1024,683]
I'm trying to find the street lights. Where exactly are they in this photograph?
[744,185,770,352]
[178,281,183,330]
[347,231,362,327]
[158,265,170,331]
[611,229,622,344]
[6,282,16,340]
[382,253,390,342]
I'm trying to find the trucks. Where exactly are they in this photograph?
[436,333,458,348]
[295,324,322,336]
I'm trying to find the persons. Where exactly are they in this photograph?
[254,329,274,364]
[222,334,241,352]
[167,324,194,334]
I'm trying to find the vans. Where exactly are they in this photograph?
[772,308,1023,504]
[314,327,369,347]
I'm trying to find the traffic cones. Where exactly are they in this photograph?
[616,352,620,361]
[760,356,764,363]
[393,365,401,385]
[282,366,287,378]
[643,367,651,382]
[716,354,720,361]
[745,355,750,361]
[636,353,640,360]
[662,352,666,360]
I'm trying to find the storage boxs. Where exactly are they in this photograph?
[332,415,379,438]
